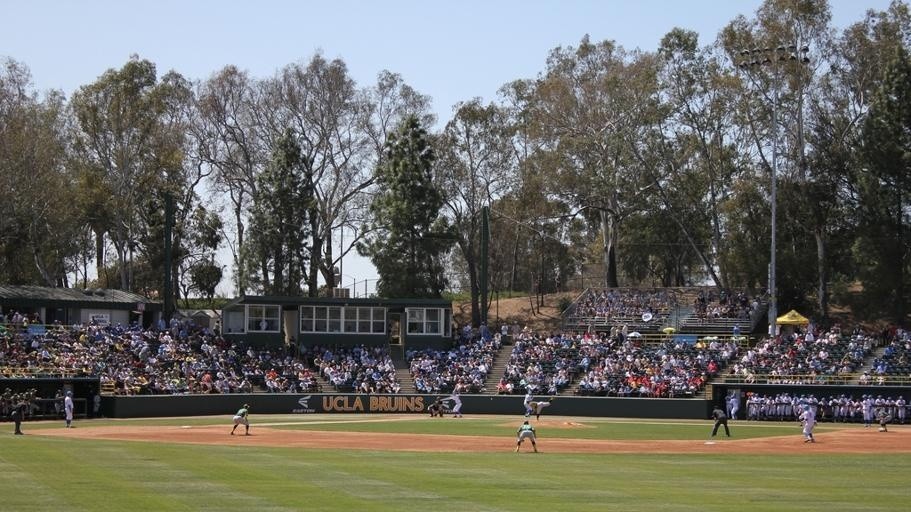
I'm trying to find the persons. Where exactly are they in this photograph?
[515,421,537,452]
[693,287,768,323]
[711,406,730,437]
[2,387,74,433]
[574,289,676,324]
[0,310,401,397]
[428,391,462,418]
[405,322,508,393]
[230,404,250,436]
[524,391,552,420]
[496,321,741,399]
[725,322,911,444]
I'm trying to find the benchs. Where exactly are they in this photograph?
[252,294,910,401]
[0,320,116,395]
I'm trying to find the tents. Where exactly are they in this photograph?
[776,309,808,333]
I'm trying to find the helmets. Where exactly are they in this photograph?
[804,404,809,410]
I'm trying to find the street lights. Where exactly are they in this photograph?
[739,44,809,337]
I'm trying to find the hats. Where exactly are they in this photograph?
[821,394,903,401]
[524,421,528,424]
[245,404,250,409]
[67,391,73,396]
[751,392,814,400]
